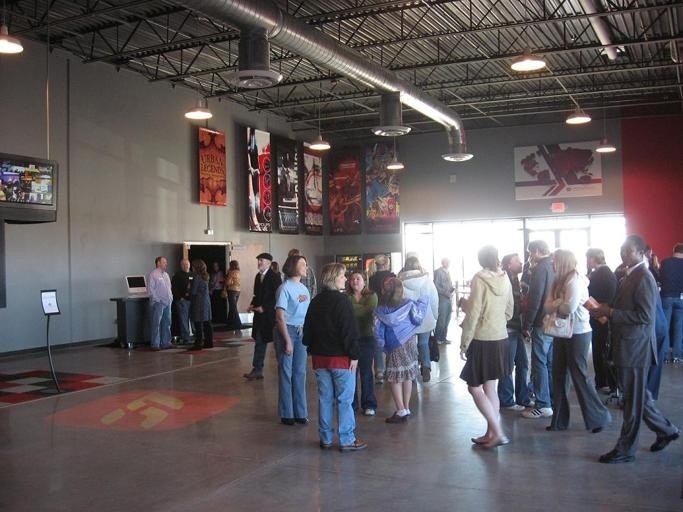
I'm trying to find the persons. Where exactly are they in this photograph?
[346,254,454,423]
[498,240,682,432]
[243,249,318,424]
[301,262,367,452]
[148,256,242,351]
[591,235,682,463]
[459,245,514,449]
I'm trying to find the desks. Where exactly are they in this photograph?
[110,297,151,349]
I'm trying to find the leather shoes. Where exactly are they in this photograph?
[599,450,635,463]
[650,429,680,451]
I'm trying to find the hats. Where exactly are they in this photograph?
[256,254,272,260]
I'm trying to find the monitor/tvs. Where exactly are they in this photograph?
[183,241,231,284]
[0,152,58,224]
[125,275,147,293]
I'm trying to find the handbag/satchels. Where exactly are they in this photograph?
[544,314,572,339]
[428,330,439,362]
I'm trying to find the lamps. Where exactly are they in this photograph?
[308,83,331,150]
[511,0,546,72]
[565,106,592,125]
[184,100,213,120]
[595,110,616,153]
[0,16,24,54]
[387,137,405,169]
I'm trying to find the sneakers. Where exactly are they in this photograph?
[339,439,366,450]
[501,400,536,411]
[364,409,375,415]
[520,408,554,418]
[386,412,408,423]
[422,366,430,382]
[281,418,307,425]
[472,434,510,448]
[243,371,258,378]
[152,341,212,351]
